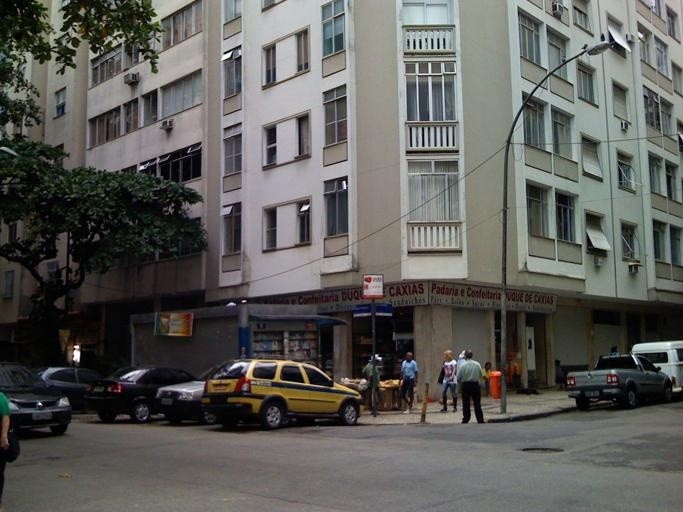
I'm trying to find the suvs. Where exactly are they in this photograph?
[0,362,73,436]
[36,367,100,414]
[201,354,365,429]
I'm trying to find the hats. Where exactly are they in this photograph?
[368,354,383,361]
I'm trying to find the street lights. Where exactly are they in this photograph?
[494,37,615,412]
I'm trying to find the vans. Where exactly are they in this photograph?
[630,340,682,396]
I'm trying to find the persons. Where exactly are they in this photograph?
[362,354,384,415]
[436,348,458,413]
[456,349,485,424]
[477,362,492,399]
[0,393,12,509]
[554,359,568,391]
[455,350,466,383]
[398,351,419,414]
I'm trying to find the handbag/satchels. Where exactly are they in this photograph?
[438,367,445,385]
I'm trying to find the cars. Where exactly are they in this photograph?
[81,364,197,426]
[154,363,220,425]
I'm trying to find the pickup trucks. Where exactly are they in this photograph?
[566,351,671,409]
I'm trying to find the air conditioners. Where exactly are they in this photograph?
[162,119,172,128]
[629,265,639,273]
[621,121,628,132]
[626,33,635,43]
[124,73,137,84]
[552,3,563,15]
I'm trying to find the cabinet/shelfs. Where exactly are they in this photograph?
[288,338,319,361]
[253,337,284,360]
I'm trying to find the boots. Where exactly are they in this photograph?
[452,396,457,412]
[440,396,447,411]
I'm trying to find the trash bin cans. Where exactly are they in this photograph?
[489,371,502,399]
